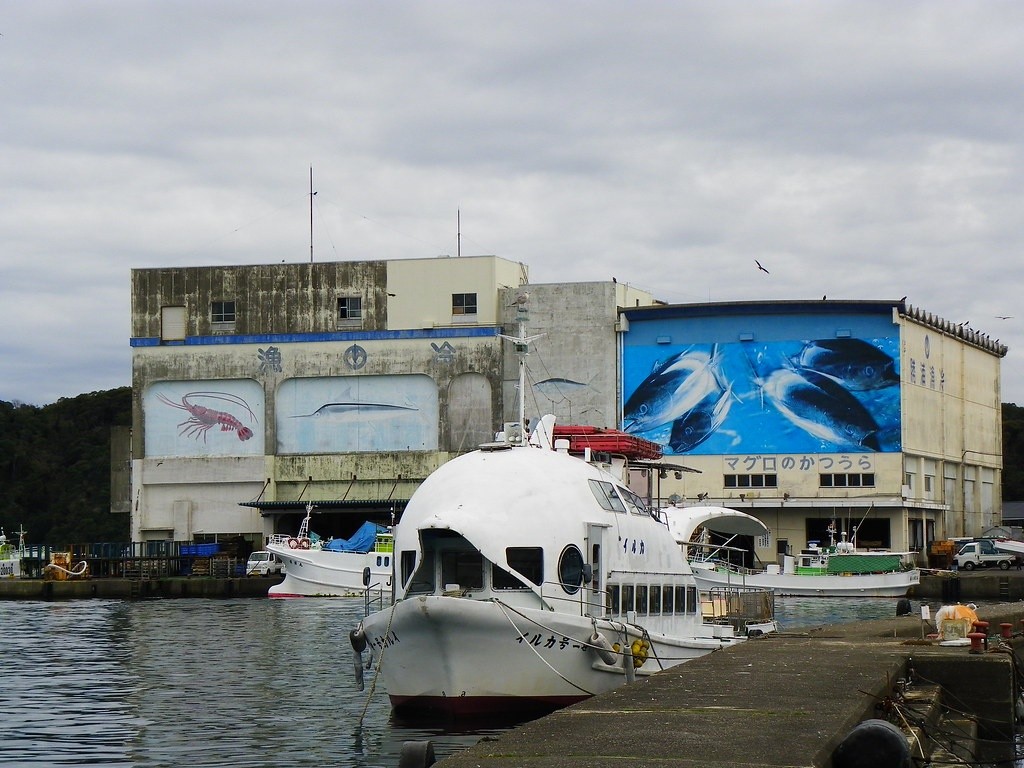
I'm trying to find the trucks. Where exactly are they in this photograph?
[246,551,282,577]
[953,542,1016,570]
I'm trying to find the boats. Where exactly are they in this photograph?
[350,293,775,724]
[654,501,920,599]
[266,499,395,601]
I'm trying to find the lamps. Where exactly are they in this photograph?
[740,494,745,502]
[659,468,682,480]
[902,484,909,501]
[697,494,705,503]
[656,335,671,345]
[836,330,851,339]
[740,333,755,341]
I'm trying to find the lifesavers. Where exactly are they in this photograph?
[300,538,311,549]
[288,539,300,549]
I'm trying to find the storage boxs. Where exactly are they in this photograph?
[928,539,955,570]
